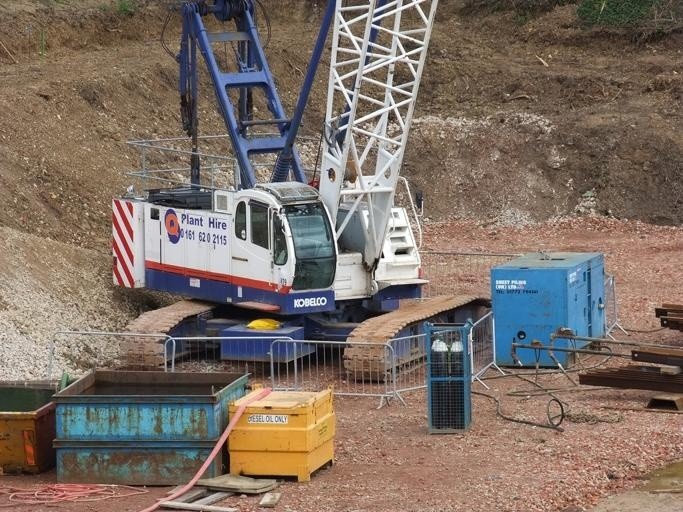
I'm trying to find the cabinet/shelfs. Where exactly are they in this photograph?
[228,383,336,483]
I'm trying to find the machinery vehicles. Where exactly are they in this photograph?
[109,0,495,383]
